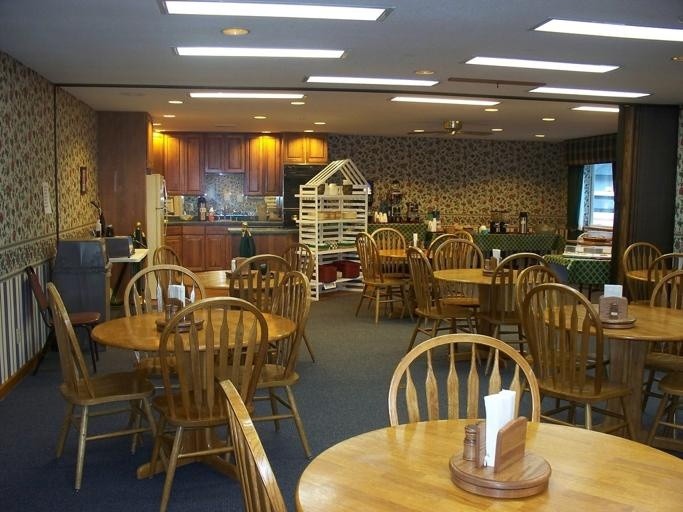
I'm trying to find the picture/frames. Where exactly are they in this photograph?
[81,167,87,194]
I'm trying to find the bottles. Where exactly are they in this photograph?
[186,197,193,215]
[209,207,214,221]
[484,257,502,270]
[231,259,237,273]
[520,212,527,234]
[463,425,478,462]
[610,304,619,320]
[165,304,181,321]
[374,212,388,224]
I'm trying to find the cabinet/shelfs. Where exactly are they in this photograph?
[99,112,328,196]
[166,221,299,272]
[297,185,370,300]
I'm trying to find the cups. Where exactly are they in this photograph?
[343,183,353,195]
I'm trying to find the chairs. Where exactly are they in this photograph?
[124,265,205,428]
[216,272,312,458]
[149,296,269,511]
[25,266,101,377]
[229,254,293,369]
[220,379,295,512]
[152,247,190,307]
[278,243,316,363]
[45,281,169,493]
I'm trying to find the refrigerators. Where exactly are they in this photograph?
[146,174,168,300]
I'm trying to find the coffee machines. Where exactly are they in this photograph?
[386,178,403,224]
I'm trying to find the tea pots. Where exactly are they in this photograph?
[324,183,340,196]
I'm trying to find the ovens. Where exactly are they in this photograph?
[282,165,328,211]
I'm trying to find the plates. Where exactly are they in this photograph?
[180,215,194,221]
[343,212,357,219]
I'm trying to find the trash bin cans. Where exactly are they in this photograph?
[53,239,111,352]
